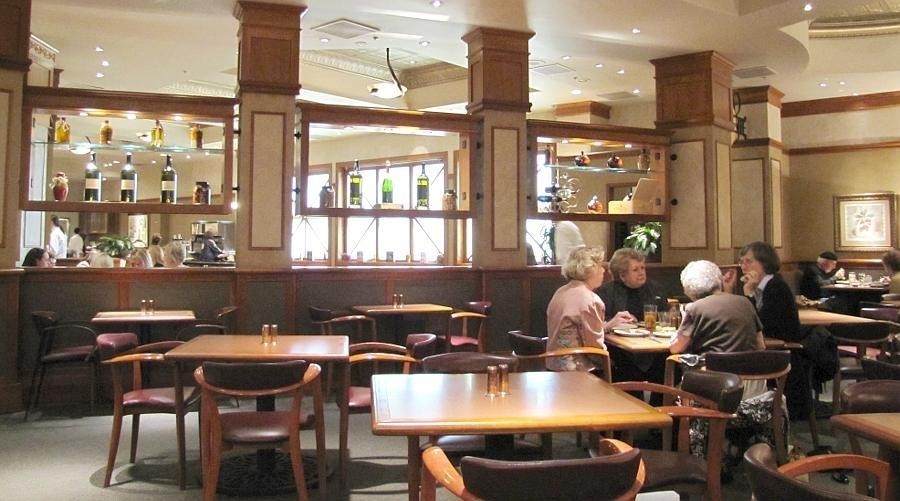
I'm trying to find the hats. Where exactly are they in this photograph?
[821,251,838,260]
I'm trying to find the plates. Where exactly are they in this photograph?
[614,328,651,337]
[835,281,849,285]
[653,332,677,339]
[869,283,885,287]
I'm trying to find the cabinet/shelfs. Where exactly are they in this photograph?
[18,83,241,215]
[295,102,483,218]
[524,118,672,223]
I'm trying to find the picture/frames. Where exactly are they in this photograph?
[832,191,899,255]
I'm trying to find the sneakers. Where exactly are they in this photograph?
[808,446,832,456]
[832,472,848,483]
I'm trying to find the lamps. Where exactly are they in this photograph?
[365,46,408,102]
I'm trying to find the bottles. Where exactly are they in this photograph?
[141,299,147,315]
[545,186,562,193]
[637,148,651,170]
[442,189,458,211]
[607,152,623,168]
[160,153,178,204]
[392,294,398,308]
[399,294,404,307]
[193,182,211,204]
[262,324,269,345]
[574,151,592,166]
[49,172,70,201]
[189,124,204,149]
[320,185,335,207]
[587,195,604,214]
[99,119,113,145]
[486,366,499,398]
[271,324,278,343]
[151,120,166,147]
[54,117,71,145]
[120,152,138,202]
[349,160,363,209]
[544,144,554,165]
[307,251,312,260]
[406,255,412,264]
[556,189,576,198]
[497,364,510,396]
[386,251,394,262]
[356,251,364,263]
[536,195,568,204]
[83,151,103,202]
[148,299,154,315]
[382,163,394,203]
[417,162,430,209]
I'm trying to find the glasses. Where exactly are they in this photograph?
[738,258,754,266]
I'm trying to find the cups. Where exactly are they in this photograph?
[642,305,659,332]
[848,270,867,287]
[658,311,673,327]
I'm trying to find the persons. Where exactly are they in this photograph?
[882,248,900,294]
[800,251,847,312]
[669,260,769,453]
[23,214,225,268]
[722,242,811,422]
[592,249,669,407]
[546,246,643,399]
